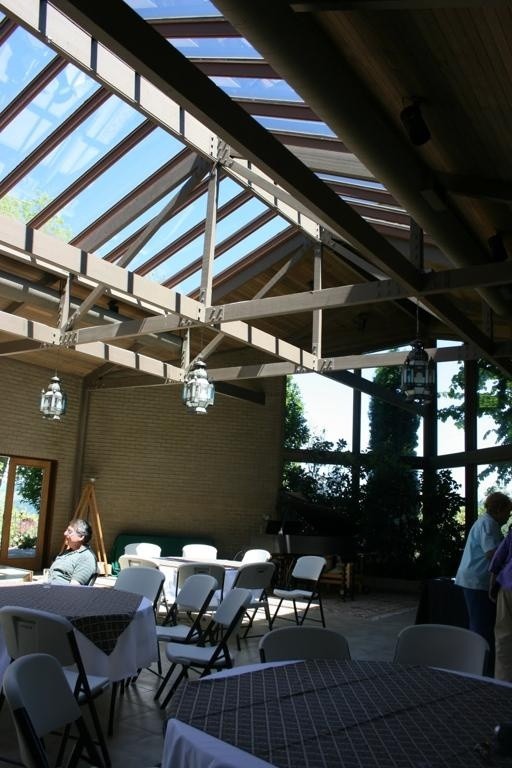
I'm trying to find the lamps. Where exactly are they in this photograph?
[40,375,67,421]
[183,361,214,416]
[401,344,438,405]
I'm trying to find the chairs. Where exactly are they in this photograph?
[113,566,165,682]
[153,588,252,710]
[0,606,119,768]
[128,557,170,627]
[443,551,463,575]
[258,626,351,664]
[125,543,163,557]
[393,624,489,677]
[226,561,276,651]
[182,544,219,561]
[155,574,218,674]
[3,653,105,768]
[271,556,328,628]
[243,549,272,563]
[84,568,100,587]
[176,561,226,648]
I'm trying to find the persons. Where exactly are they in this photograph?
[454,492,512,679]
[50,519,96,586]
[489,523,512,683]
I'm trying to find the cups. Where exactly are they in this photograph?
[22,569,34,583]
[43,568,54,589]
